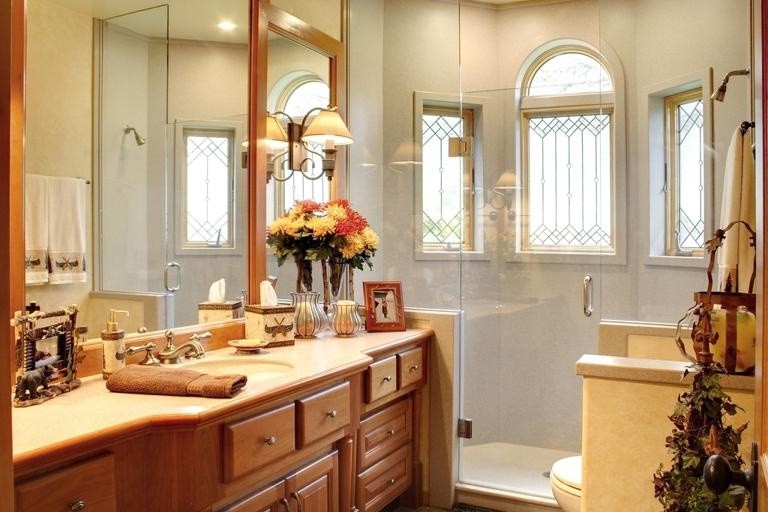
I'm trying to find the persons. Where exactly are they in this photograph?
[382,299,389,319]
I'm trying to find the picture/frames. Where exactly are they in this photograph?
[361,280,409,334]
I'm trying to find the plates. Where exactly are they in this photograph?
[227,339,269,348]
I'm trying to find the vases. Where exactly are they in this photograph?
[327,254,346,303]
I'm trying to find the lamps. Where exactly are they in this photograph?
[709,69,749,102]
[287,98,354,185]
[296,254,314,293]
[242,108,291,183]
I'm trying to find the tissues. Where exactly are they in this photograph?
[197,278,242,330]
[243,280,296,347]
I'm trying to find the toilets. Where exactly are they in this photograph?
[548,447,584,512]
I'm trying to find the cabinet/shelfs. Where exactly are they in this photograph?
[150,374,353,512]
[353,346,425,512]
[15,440,155,511]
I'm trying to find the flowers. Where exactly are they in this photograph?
[304,199,380,292]
[266,199,314,292]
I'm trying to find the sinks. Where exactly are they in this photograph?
[174,354,299,387]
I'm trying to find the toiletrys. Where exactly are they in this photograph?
[100,308,130,382]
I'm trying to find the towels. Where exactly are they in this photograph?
[102,362,248,398]
[48,177,88,285]
[25,172,47,287]
[715,119,754,295]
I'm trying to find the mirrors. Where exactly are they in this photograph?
[3,0,255,385]
[257,4,348,304]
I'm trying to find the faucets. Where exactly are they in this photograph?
[158,330,204,365]
